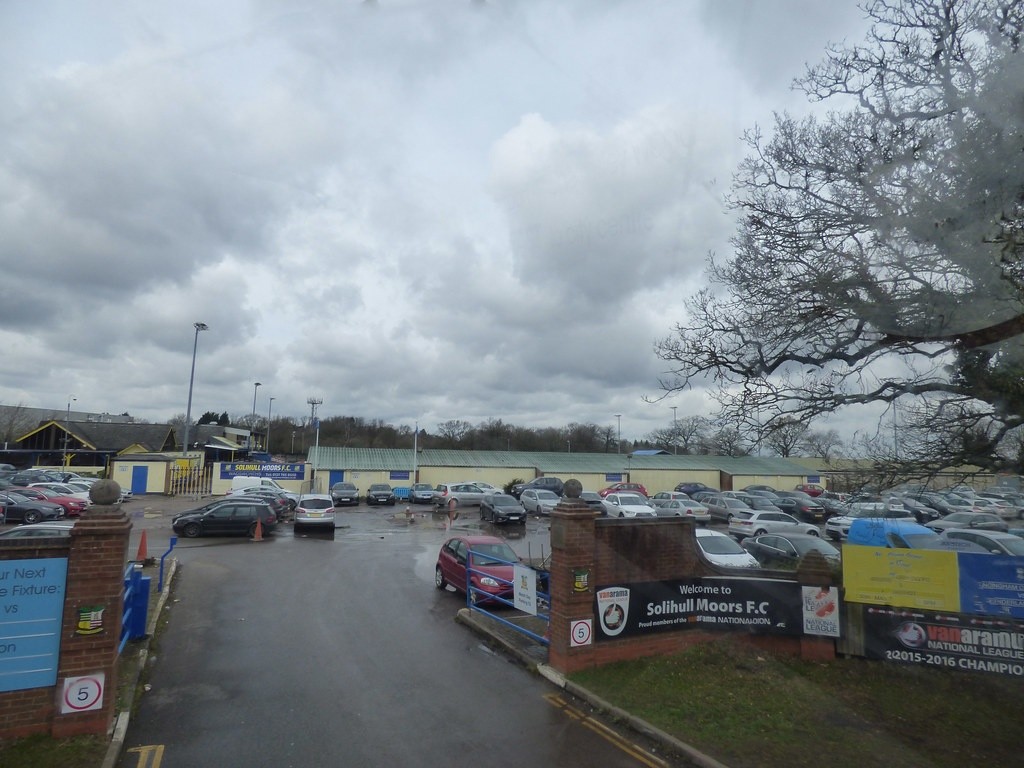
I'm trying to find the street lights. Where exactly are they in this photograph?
[567,440,570,453]
[670,406,678,456]
[614,414,624,455]
[181,322,211,456]
[62,394,78,475]
[266,397,275,451]
[253,382,262,416]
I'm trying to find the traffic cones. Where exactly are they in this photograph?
[249,516,266,542]
[136,527,148,559]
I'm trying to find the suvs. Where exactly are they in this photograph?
[434,481,504,508]
[171,498,279,538]
[408,483,434,503]
[510,477,565,499]
[294,493,337,534]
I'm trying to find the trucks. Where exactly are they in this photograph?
[226,476,300,505]
[847,518,943,552]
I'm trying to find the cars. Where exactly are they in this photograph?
[434,534,524,606]
[366,482,396,506]
[479,492,527,524]
[330,482,360,506]
[582,474,1024,571]
[520,489,562,515]
[0,490,65,525]
[224,486,291,516]
[0,464,135,511]
[3,486,88,518]
